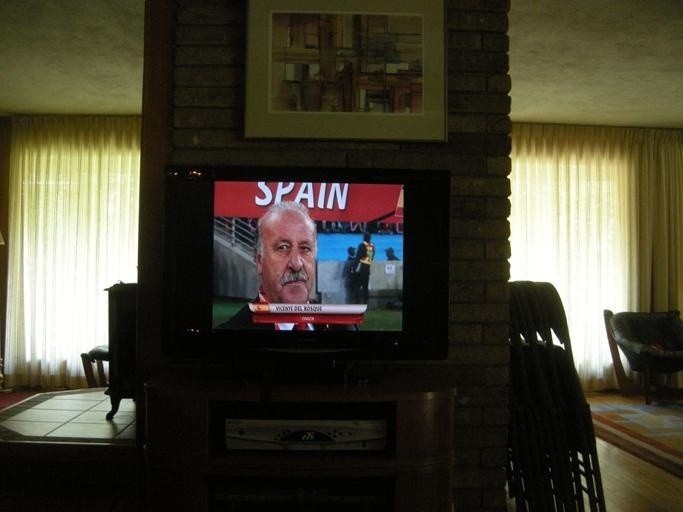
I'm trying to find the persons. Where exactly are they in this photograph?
[385,247,398,261]
[352,232,375,304]
[216,201,359,332]
[341,248,357,304]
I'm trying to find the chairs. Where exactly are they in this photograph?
[604,308,683,406]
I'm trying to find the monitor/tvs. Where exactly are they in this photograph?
[161,163,452,362]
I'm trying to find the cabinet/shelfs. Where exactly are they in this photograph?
[142,363,458,512]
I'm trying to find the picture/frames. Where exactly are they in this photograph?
[242,0,447,144]
